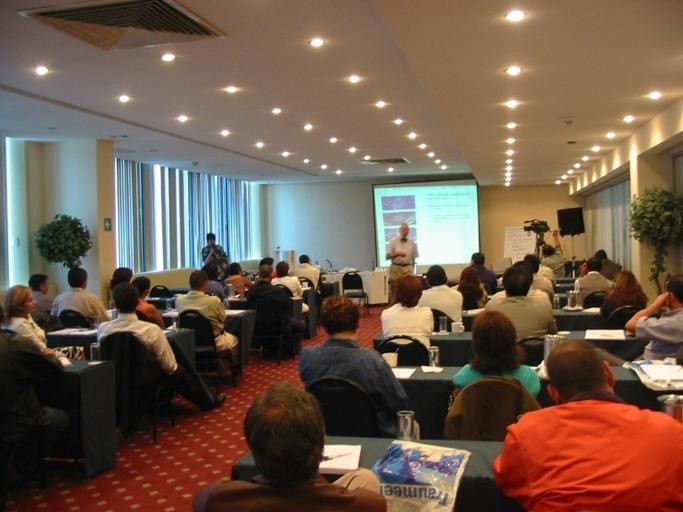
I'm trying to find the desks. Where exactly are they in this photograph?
[414,273,607,332]
[373,329,649,366]
[390,366,682,439]
[232,435,681,508]
[0,279,341,481]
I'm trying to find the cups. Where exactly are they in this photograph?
[438,315,448,333]
[397,409,414,440]
[226,284,232,297]
[656,394,682,422]
[164,297,179,334]
[543,289,576,383]
[427,345,441,369]
[90,343,99,361]
[105,309,119,318]
[300,282,307,288]
[451,322,465,335]
[381,352,398,369]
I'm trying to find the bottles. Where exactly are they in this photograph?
[52,346,86,360]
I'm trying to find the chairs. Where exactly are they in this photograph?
[343,271,369,320]
[303,375,378,437]
[516,337,545,365]
[373,335,431,367]
[608,305,640,332]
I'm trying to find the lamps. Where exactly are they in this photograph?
[555,206,585,278]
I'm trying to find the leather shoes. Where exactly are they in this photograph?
[212,391,227,408]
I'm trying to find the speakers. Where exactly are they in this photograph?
[556,206,586,237]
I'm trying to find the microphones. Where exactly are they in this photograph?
[373,260,374,271]
[326,259,332,272]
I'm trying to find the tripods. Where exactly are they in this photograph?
[533,233,545,262]
[567,235,579,277]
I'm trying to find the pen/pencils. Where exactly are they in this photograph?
[323,452,351,461]
